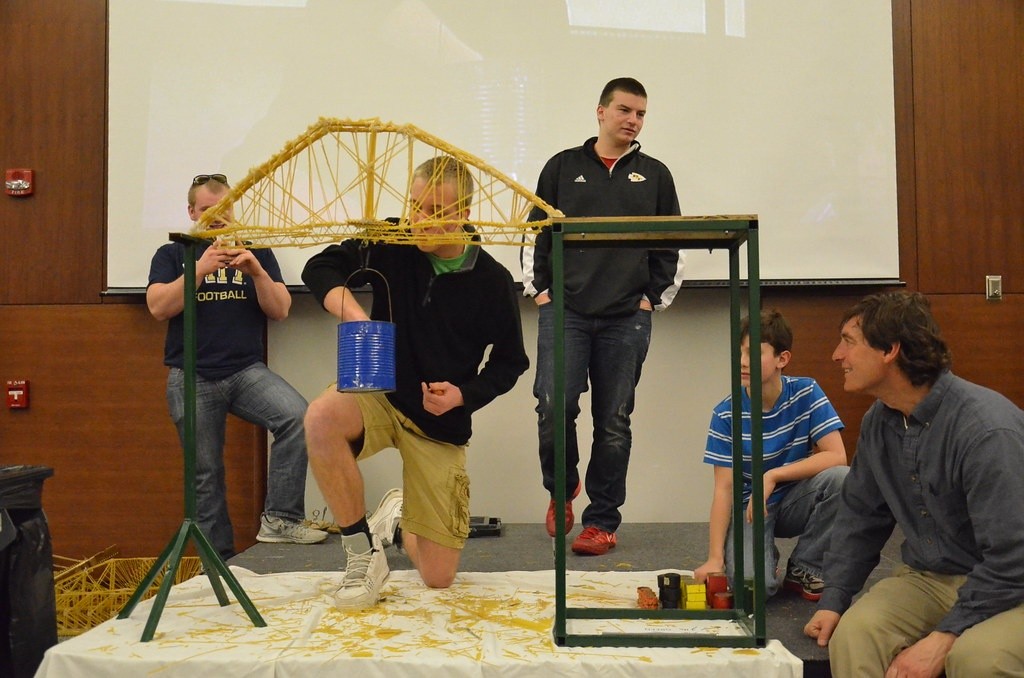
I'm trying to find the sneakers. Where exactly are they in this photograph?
[546,478,582,536]
[366,486,403,548]
[573,523,617,555]
[785,557,825,602]
[333,532,390,610]
[256,515,328,543]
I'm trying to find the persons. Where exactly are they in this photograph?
[803,288,1024,677]
[520,76,686,555]
[300,156,529,608]
[146,175,329,571]
[694,308,851,601]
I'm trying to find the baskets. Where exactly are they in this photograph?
[54,557,208,636]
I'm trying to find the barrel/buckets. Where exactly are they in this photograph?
[337,265,395,395]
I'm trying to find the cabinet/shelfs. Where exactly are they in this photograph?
[550,214,767,649]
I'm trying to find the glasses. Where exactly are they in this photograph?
[192,174,228,185]
